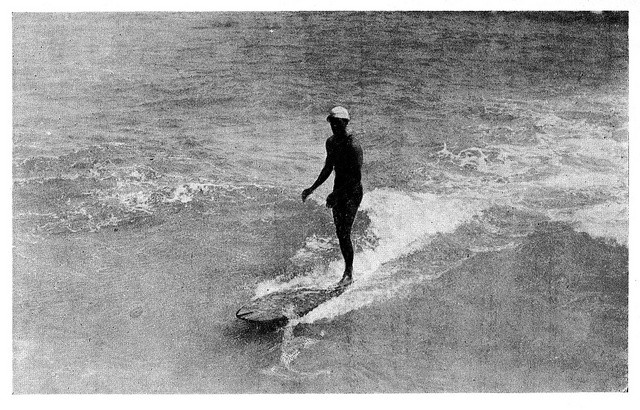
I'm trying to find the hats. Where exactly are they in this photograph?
[327,106,350,120]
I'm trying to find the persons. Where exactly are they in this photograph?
[301,106,363,285]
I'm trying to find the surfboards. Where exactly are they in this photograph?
[236,287,351,324]
